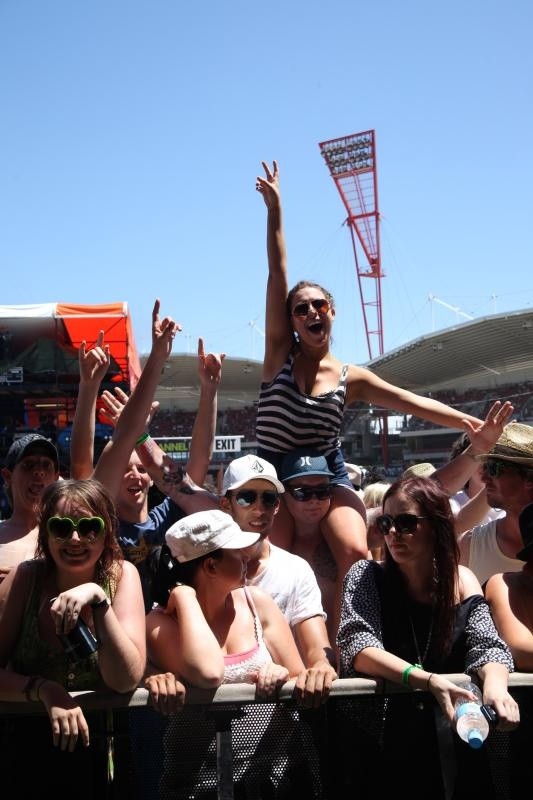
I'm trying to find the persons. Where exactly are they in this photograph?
[0,161,533,799]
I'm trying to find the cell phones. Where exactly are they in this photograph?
[481,704,499,722]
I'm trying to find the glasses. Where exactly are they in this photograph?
[374,513,432,536]
[483,464,519,479]
[46,516,104,544]
[20,460,55,471]
[284,483,333,502]
[232,490,278,507]
[291,299,331,319]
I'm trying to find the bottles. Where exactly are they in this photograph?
[50,596,98,658]
[453,681,489,748]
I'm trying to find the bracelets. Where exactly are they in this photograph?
[90,597,111,609]
[25,676,46,702]
[427,673,434,690]
[136,432,151,447]
[402,664,422,691]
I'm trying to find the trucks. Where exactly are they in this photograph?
[0,300,141,461]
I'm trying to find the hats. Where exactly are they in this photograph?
[279,456,335,482]
[165,509,260,563]
[477,421,533,462]
[344,462,365,497]
[6,434,59,469]
[401,462,436,481]
[219,453,285,496]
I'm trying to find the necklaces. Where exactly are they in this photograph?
[409,598,433,665]
